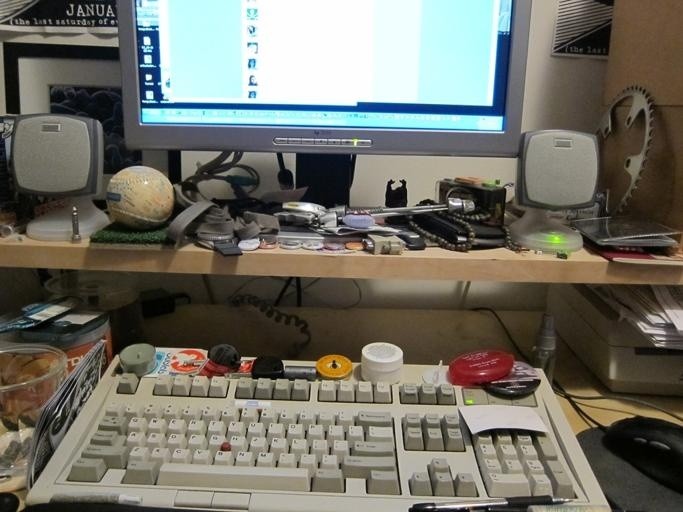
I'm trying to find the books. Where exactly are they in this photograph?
[26,338,108,492]
[569,214,682,248]
[581,242,683,265]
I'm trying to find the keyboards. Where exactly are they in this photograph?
[23,342,614,511]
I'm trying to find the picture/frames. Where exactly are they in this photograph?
[3,41,182,209]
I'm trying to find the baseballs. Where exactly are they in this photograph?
[106,166,175,229]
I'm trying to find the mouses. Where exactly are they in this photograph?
[603,414,683,495]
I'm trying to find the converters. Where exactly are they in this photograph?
[141,286,176,318]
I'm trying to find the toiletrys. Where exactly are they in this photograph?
[529,313,557,389]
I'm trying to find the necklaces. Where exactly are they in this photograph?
[405,198,490,252]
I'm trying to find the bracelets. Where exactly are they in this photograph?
[502,223,522,253]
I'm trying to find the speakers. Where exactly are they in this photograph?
[10,112,110,241]
[507,128,601,255]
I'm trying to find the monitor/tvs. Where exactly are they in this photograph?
[113,0,533,246]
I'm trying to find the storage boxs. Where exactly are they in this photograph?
[605,0,683,231]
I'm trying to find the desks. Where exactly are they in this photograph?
[0,206,683,512]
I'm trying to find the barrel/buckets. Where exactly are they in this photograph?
[1,344,67,494]
